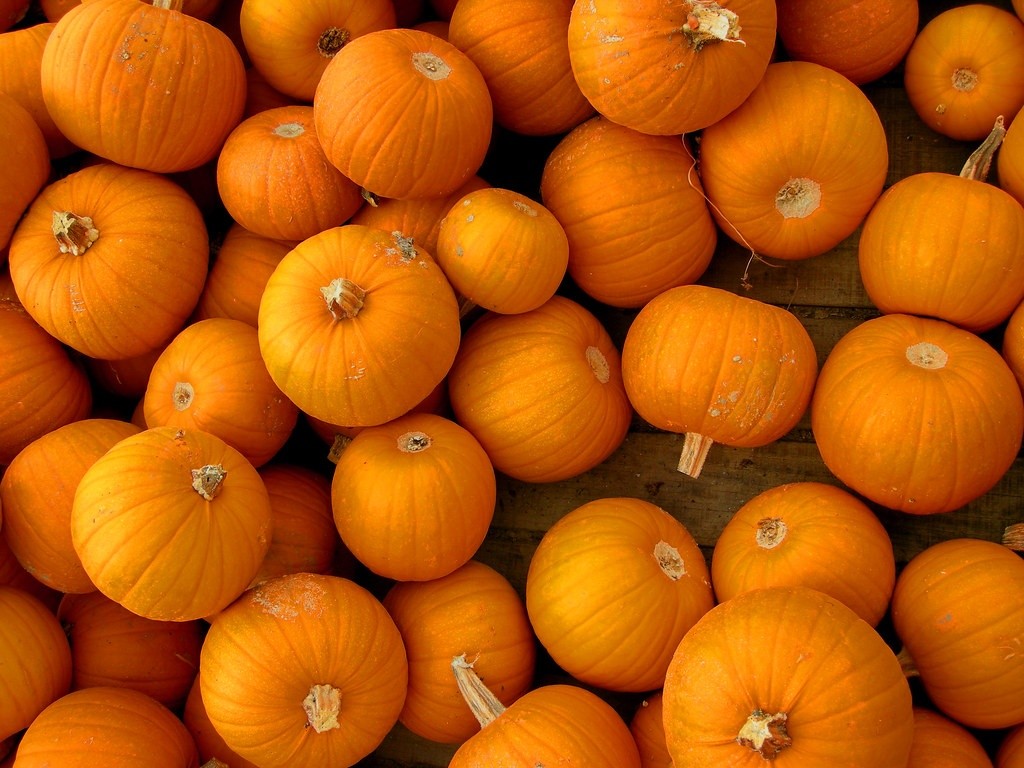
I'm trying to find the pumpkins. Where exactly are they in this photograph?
[0,0,1024,768]
